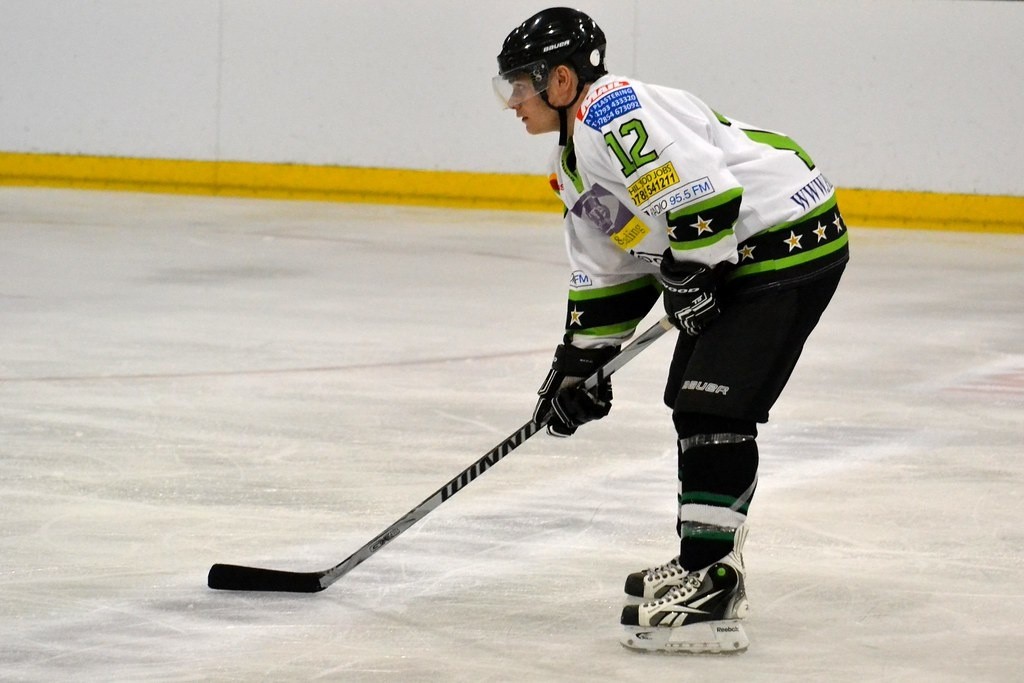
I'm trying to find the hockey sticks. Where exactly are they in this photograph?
[206,314,673,594]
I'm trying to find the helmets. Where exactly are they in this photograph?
[493,7,607,109]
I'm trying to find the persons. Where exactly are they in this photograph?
[491,7,851,656]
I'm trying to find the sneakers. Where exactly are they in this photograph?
[625,529,751,619]
[619,524,750,653]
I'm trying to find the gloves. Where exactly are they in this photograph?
[533,344,621,437]
[660,247,725,335]
